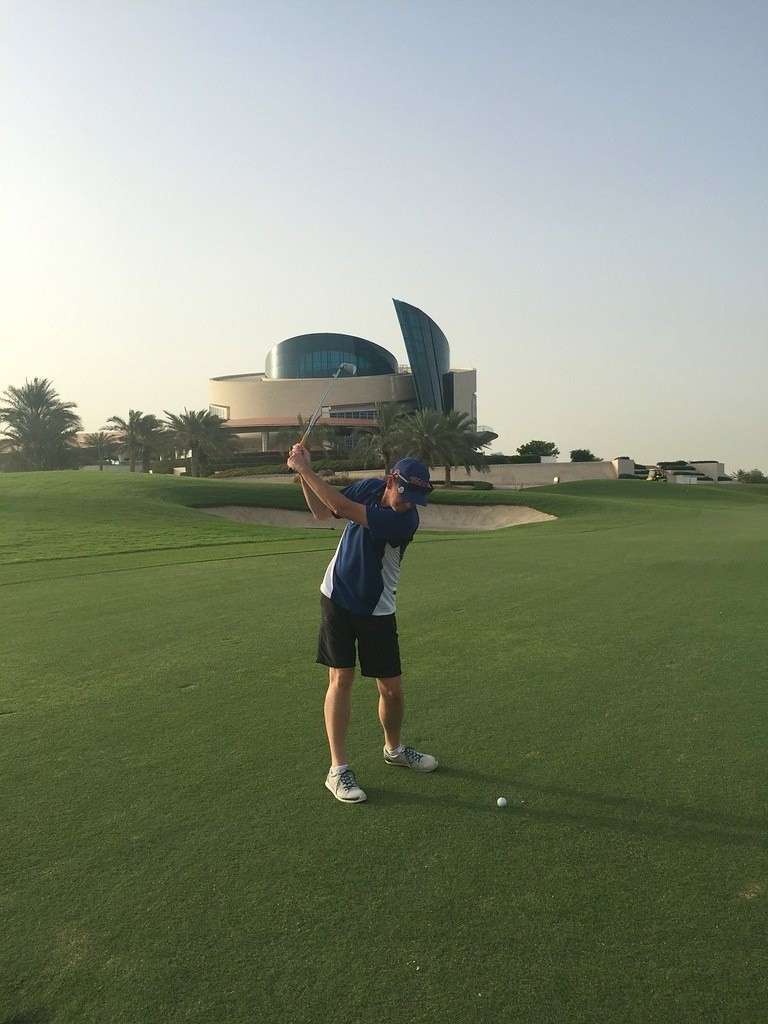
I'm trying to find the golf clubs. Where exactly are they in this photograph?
[287,363,357,475]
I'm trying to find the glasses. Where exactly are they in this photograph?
[393,472,434,496]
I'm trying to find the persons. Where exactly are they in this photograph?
[658,470,661,476]
[664,475,667,482]
[287,442,438,803]
[654,472,657,477]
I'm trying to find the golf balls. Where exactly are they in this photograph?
[496,797,506,807]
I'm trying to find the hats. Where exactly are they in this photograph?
[391,456,430,507]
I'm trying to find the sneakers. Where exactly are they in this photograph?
[384,744,438,772]
[324,765,367,804]
